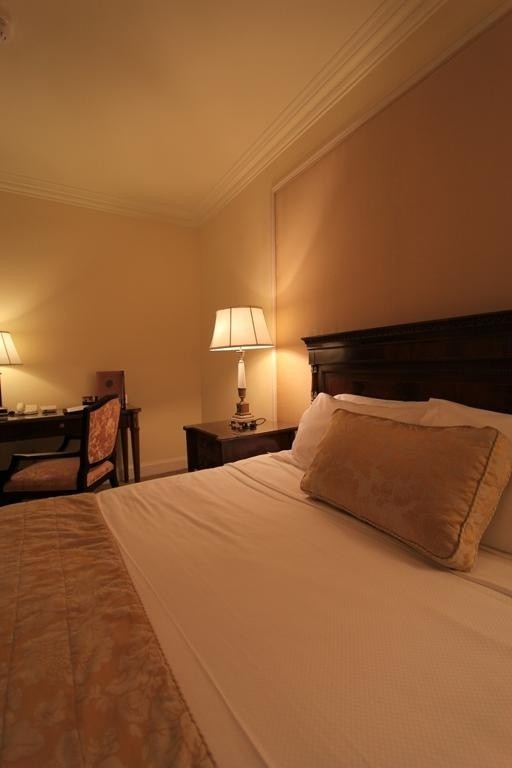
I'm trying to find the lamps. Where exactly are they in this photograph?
[0,330,25,416]
[209,304,276,429]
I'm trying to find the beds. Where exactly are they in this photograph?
[1,309,512,768]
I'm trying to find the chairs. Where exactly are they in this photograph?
[1,394,121,495]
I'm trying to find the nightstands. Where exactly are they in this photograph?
[183,419,298,472]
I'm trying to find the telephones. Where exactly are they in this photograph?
[17,402,57,415]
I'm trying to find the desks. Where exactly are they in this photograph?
[0,405,143,484]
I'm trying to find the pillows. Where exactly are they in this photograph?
[420,398,510,554]
[267,392,426,471]
[333,393,429,408]
[300,408,509,573]
